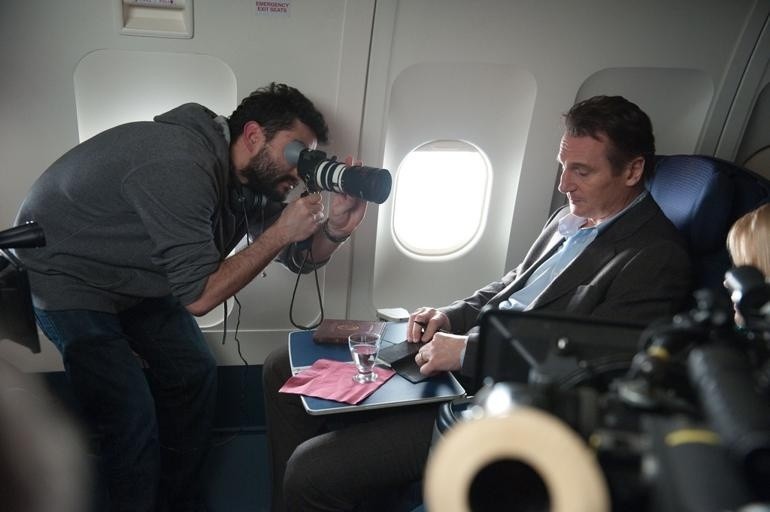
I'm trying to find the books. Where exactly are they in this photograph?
[375,334,440,385]
[311,317,387,348]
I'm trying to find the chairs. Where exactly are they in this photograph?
[644,155,769,279]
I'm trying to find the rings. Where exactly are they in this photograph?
[419,351,427,363]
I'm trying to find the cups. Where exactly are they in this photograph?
[348,331,382,384]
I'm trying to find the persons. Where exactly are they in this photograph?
[1,357,101,511]
[725,199,769,348]
[10,75,372,512]
[258,92,709,512]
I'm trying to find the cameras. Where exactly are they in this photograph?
[296,147,392,204]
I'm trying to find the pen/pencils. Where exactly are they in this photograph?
[413,321,455,334]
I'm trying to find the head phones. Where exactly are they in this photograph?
[228,173,267,214]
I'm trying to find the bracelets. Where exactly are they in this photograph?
[323,217,352,243]
